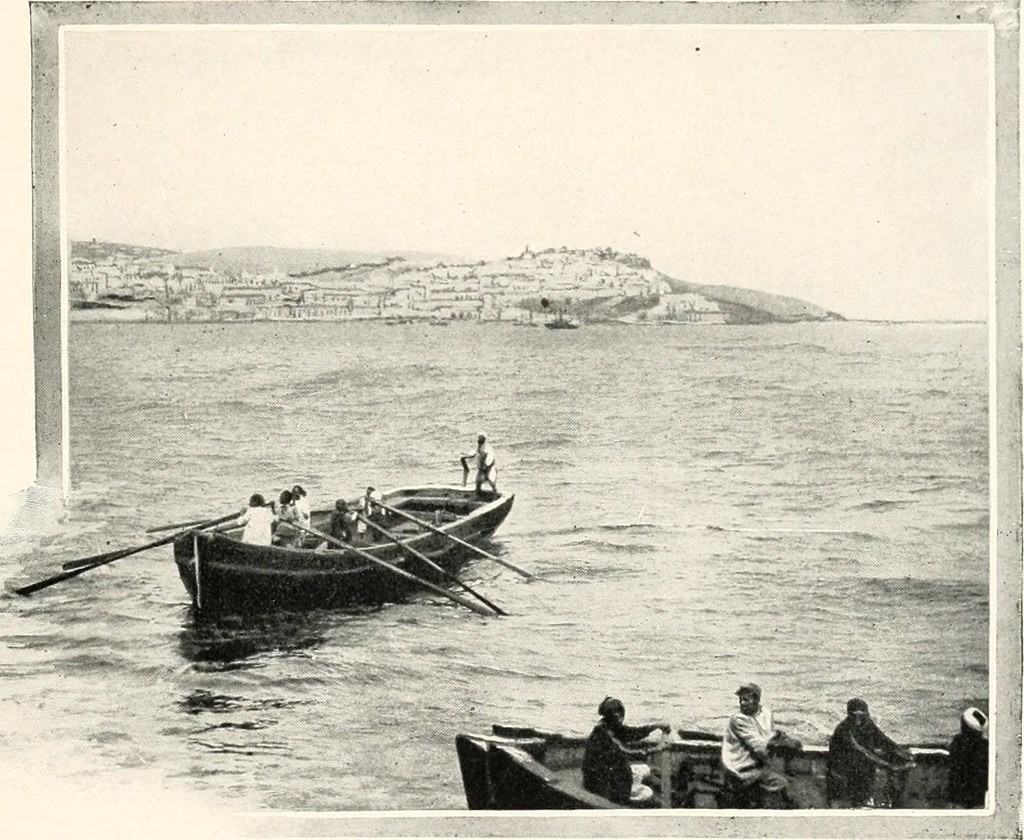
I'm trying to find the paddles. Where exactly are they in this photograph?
[283,518,496,617]
[13,512,243,596]
[60,544,136,571]
[144,517,213,534]
[350,507,508,616]
[660,734,673,808]
[372,497,535,580]
[677,728,724,743]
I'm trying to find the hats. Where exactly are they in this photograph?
[735,683,761,698]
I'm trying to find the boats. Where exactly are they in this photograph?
[452,719,989,809]
[172,482,517,619]
[543,320,582,330]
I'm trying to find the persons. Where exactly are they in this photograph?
[948,708,990,807]
[581,697,672,805]
[237,493,272,544]
[828,698,917,808]
[719,683,801,802]
[356,485,379,539]
[328,499,352,549]
[290,485,310,526]
[464,433,497,495]
[270,490,303,524]
